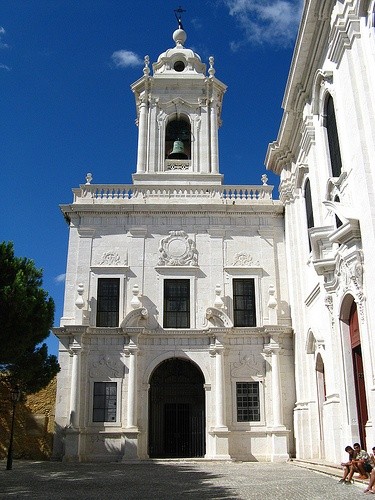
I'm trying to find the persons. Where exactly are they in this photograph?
[364,447,375,493]
[352,443,373,479]
[337,445,358,484]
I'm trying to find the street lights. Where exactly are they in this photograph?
[5,387,23,471]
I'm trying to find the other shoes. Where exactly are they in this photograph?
[363,489,374,494]
[337,479,345,484]
[343,480,351,484]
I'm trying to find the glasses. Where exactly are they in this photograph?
[354,446,358,449]
